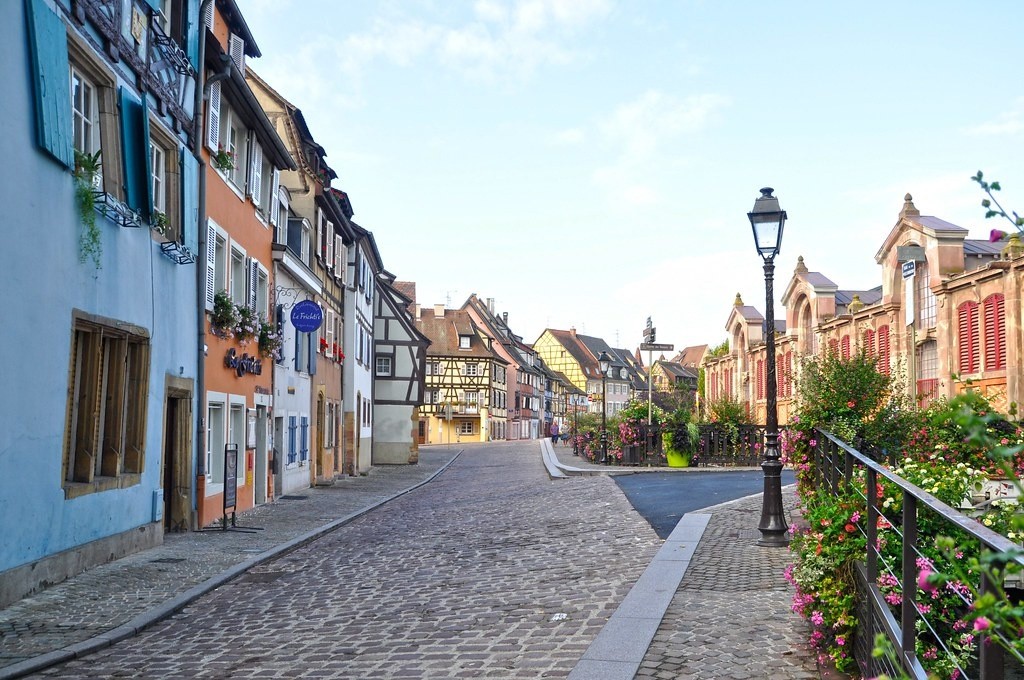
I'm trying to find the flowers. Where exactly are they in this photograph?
[215,141,240,173]
[565,400,762,470]
[154,207,171,236]
[209,291,284,362]
[332,342,346,364]
[319,337,329,354]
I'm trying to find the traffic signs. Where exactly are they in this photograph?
[640,316,674,351]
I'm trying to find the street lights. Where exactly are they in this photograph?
[596,351,611,464]
[571,387,581,456]
[748,186,791,549]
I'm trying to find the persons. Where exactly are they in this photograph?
[560,420,570,448]
[550,420,560,449]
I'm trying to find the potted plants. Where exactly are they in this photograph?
[85,147,104,188]
